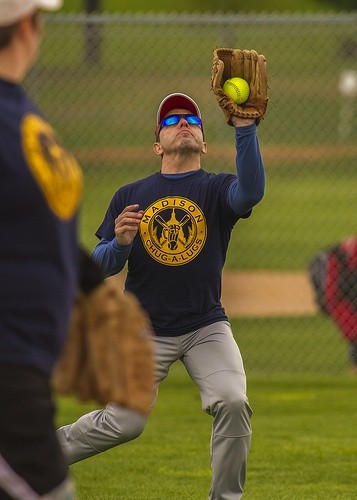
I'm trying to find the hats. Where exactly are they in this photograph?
[1,0,63,27]
[155,91,202,135]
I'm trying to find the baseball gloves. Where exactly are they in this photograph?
[53,284,155,416]
[210,48,276,127]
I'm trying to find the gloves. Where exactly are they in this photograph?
[210,47,269,125]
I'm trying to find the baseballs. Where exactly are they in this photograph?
[223,77,250,104]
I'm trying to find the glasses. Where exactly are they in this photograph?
[158,114,203,133]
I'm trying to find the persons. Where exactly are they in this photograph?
[56,47,269,500]
[0,0,154,500]
